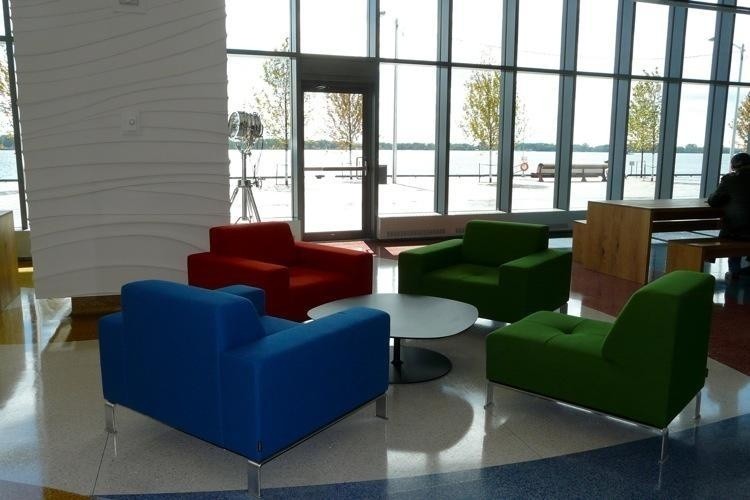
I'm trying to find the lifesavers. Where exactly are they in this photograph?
[520,163,529,171]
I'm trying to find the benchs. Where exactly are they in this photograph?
[532,163,608,182]
[666,239,728,272]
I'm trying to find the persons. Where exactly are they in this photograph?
[708,153,749,271]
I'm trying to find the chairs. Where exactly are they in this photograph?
[188,221,373,322]
[485,269,715,462]
[399,220,572,326]
[97,279,390,497]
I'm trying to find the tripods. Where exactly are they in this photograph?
[230,151,261,224]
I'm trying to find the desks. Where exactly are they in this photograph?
[572,199,714,284]
